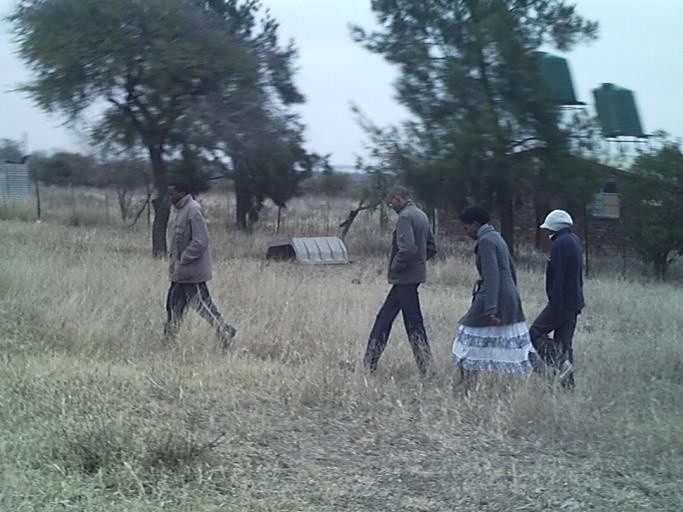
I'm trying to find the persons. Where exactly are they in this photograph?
[451,206,559,400]
[528,209,583,396]
[360,185,439,383]
[157,178,236,357]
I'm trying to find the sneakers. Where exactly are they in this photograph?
[557,360,575,381]
[219,324,237,349]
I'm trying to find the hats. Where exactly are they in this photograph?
[539,210,573,232]
